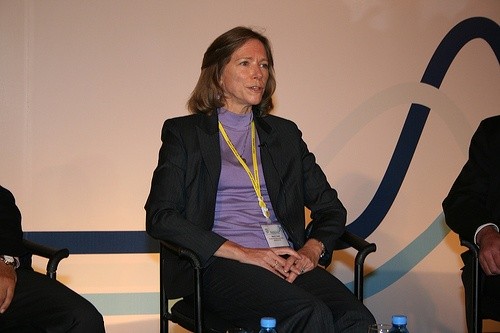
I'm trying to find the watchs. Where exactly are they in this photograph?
[0,254,17,267]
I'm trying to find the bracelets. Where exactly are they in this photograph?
[318,241,325,257]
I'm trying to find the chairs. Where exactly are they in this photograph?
[17,239,69,280]
[156,234,377,333]
[460,239,500,333]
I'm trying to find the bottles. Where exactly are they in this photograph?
[388,315,410,333]
[258,317,277,333]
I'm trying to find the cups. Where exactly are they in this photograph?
[367,324,392,333]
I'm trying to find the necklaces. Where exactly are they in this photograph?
[221,121,250,165]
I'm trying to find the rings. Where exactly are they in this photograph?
[272,260,278,267]
[301,269,303,272]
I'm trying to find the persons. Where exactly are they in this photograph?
[0,185,104,333]
[441,113,500,333]
[144,26,379,333]
